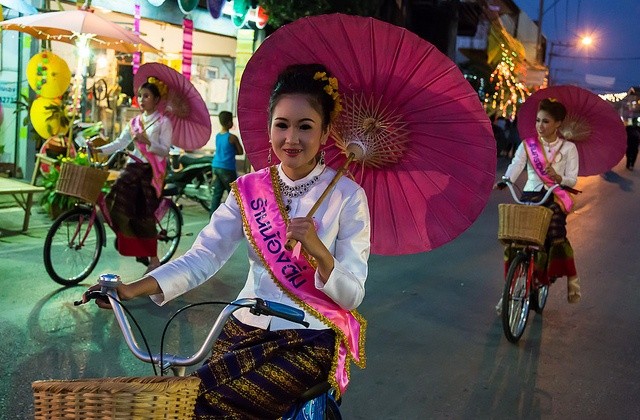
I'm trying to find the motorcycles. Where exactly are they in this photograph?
[165,154,229,213]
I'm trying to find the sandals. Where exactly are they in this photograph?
[567,278,582,303]
[496,293,504,317]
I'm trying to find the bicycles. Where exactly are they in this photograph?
[492,175,582,344]
[31,275,341,420]
[43,137,184,292]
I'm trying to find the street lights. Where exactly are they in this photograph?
[548,34,593,85]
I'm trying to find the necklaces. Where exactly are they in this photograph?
[276,164,328,197]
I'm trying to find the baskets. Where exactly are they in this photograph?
[32,376,201,420]
[498,204,554,246]
[56,162,110,204]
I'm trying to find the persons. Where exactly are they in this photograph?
[81,63,371,420]
[489,111,521,160]
[490,98,582,316]
[81,81,174,276]
[625,117,640,170]
[209,111,243,218]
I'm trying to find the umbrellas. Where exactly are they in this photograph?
[0,0,165,158]
[516,84,627,176]
[235,12,499,257]
[133,62,212,150]
